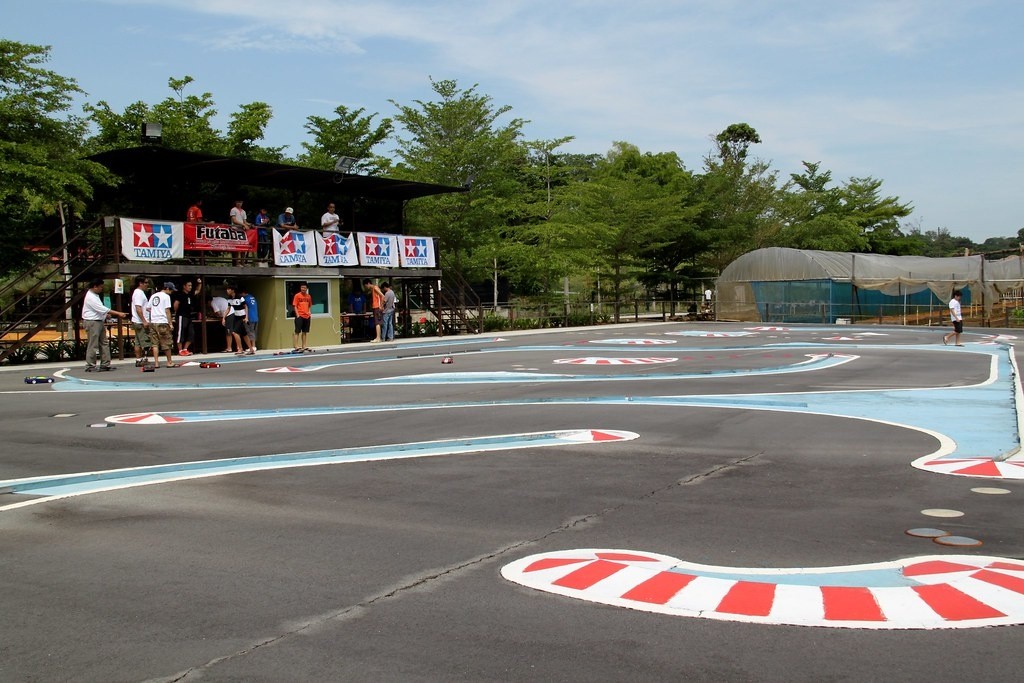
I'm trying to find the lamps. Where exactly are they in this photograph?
[141,123,162,146]
[461,174,475,187]
[333,156,358,173]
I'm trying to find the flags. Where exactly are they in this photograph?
[272,231,436,268]
[188,221,257,249]
[119,218,184,263]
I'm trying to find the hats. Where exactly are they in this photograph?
[167,282,178,292]
[285,207,294,214]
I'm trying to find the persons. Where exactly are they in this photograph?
[704,286,713,309]
[205,285,259,357]
[81,273,130,373]
[255,206,271,226]
[278,207,300,228]
[321,202,341,238]
[347,278,395,343]
[187,196,203,222]
[132,275,202,369]
[290,283,313,353]
[230,195,255,231]
[943,290,963,346]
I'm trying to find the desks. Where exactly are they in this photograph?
[340,312,374,343]
[193,319,219,322]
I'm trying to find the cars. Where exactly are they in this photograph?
[200,361,220,369]
[136,358,149,367]
[143,364,155,373]
[178,349,194,356]
[441,356,454,364]
[24,375,54,385]
[273,348,314,354]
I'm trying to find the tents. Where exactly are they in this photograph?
[715,247,1024,326]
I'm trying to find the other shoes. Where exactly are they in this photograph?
[136,359,154,367]
[87,367,101,372]
[179,349,193,356]
[100,365,117,371]
[370,338,381,343]
[943,336,947,345]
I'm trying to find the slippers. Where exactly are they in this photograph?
[155,365,161,368]
[303,347,312,351]
[235,351,244,354]
[167,364,180,367]
[221,350,232,353]
[245,351,255,355]
[296,348,304,352]
